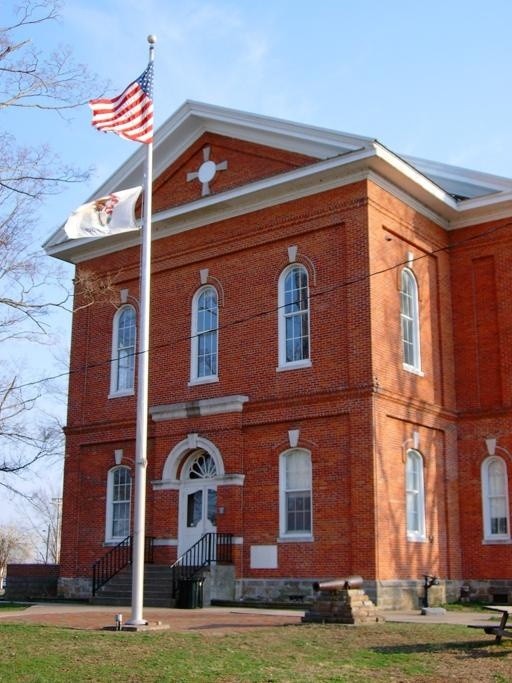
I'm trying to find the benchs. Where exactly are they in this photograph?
[467,624,512,638]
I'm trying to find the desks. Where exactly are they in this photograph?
[482,605,512,646]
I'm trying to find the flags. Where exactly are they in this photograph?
[87,60,155,145]
[62,185,143,240]
